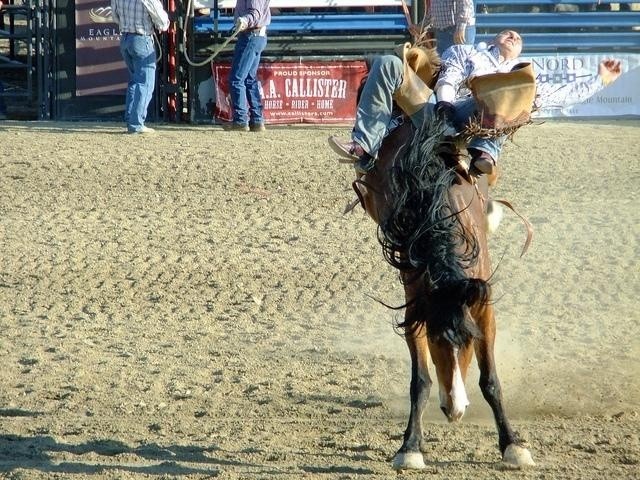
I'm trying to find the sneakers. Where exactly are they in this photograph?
[222,122,266,133]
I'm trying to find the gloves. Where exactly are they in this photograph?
[235,16,248,32]
[435,101,458,137]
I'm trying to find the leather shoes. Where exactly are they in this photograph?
[138,126,155,134]
[328,135,372,166]
[469,151,495,181]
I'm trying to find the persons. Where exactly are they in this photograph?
[0,0,10,122]
[406,0,478,61]
[325,26,624,176]
[221,0,273,134]
[109,0,172,136]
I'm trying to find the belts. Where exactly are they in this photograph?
[239,27,261,33]
[121,32,151,37]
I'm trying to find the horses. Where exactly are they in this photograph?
[357,38,538,470]
